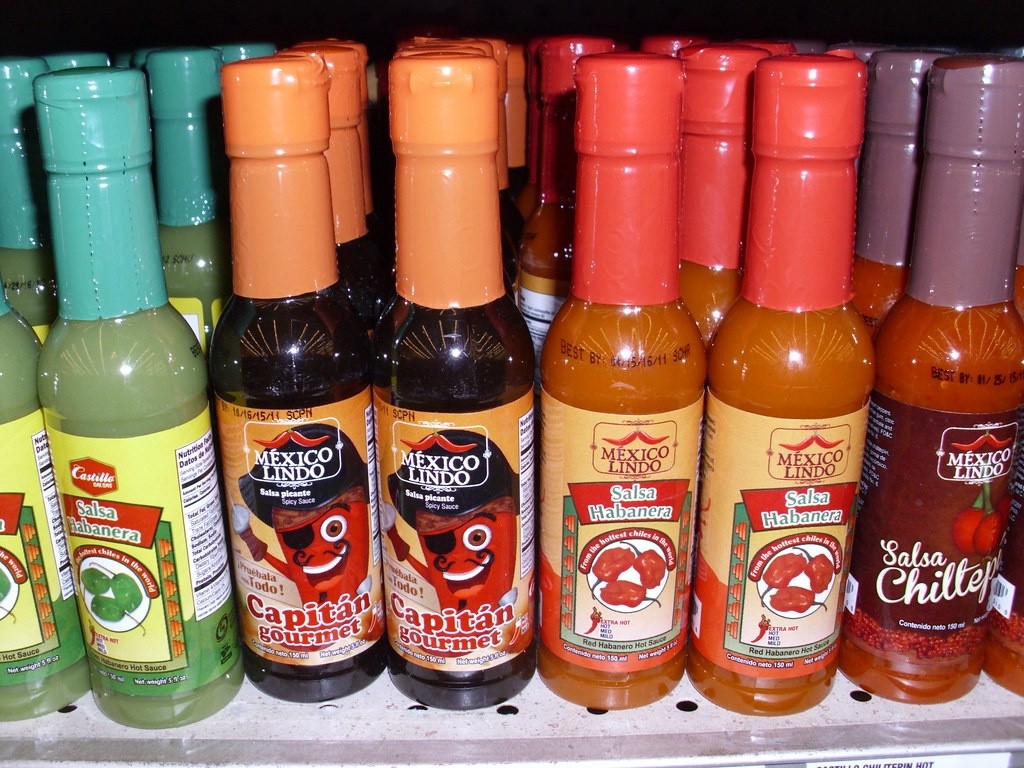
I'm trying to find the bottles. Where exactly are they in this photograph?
[0,34,1024,731]
